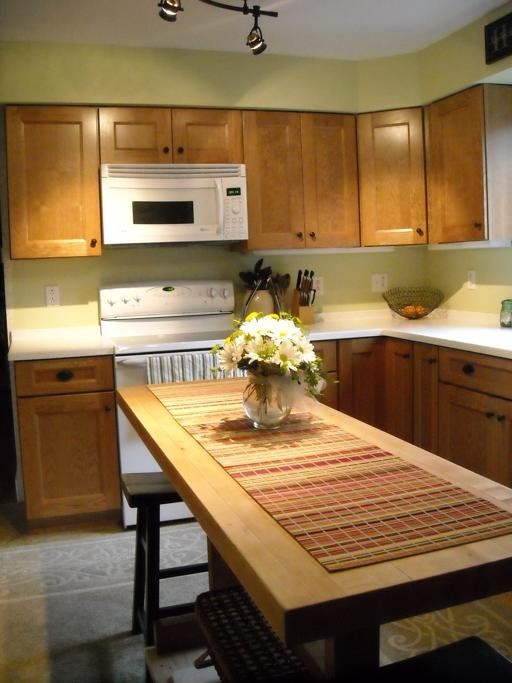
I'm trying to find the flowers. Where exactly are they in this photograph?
[210,309,332,410]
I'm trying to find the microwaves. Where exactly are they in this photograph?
[99,163,248,247]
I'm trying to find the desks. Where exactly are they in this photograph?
[114,368,511,683]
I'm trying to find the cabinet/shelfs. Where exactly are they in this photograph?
[379,336,439,455]
[426,82,512,249]
[242,105,361,249]
[335,333,379,433]
[6,102,106,259]
[99,102,245,167]
[311,336,336,409]
[353,105,428,246]
[440,344,512,488]
[9,355,119,527]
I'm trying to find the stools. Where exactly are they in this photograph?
[118,465,239,646]
[366,634,511,682]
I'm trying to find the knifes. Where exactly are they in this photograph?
[296,268,317,306]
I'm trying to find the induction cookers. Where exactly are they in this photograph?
[104,330,238,356]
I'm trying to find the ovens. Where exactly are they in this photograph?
[114,356,249,528]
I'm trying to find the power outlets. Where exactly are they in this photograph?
[46,285,60,309]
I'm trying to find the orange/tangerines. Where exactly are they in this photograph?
[403,305,424,317]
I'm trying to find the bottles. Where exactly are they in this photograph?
[499,299,511,329]
[241,289,274,318]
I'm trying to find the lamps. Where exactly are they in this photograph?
[157,0,268,57]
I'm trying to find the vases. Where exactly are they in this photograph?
[239,368,301,427]
